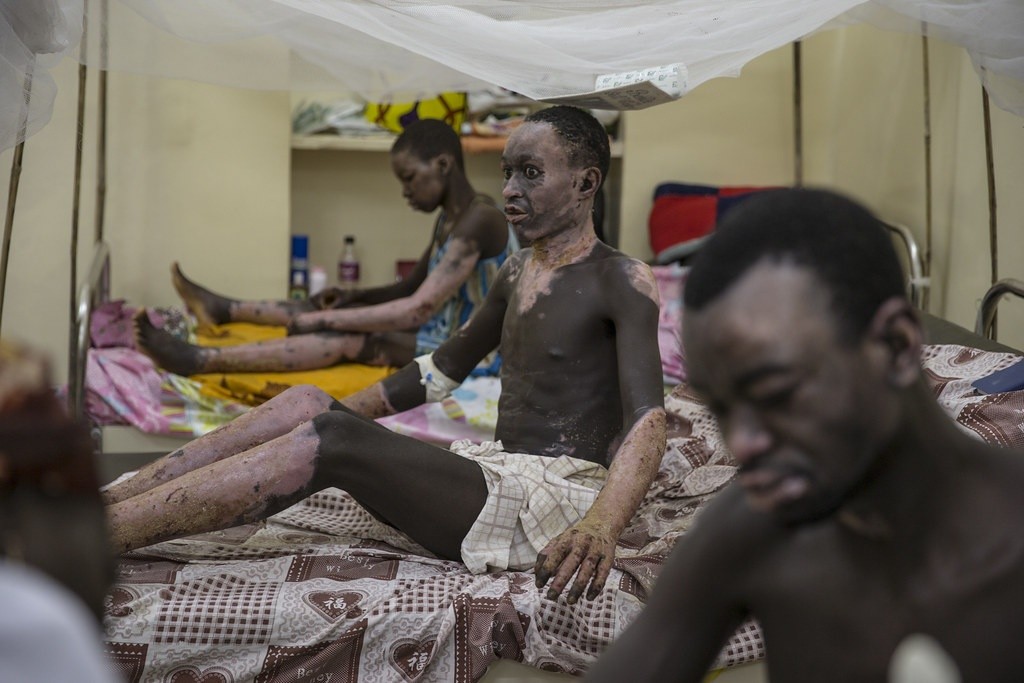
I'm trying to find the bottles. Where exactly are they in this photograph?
[337,235,361,291]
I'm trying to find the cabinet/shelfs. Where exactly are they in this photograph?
[289,83,622,304]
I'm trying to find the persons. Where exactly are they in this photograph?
[135,118,514,376]
[478,187,1024,683]
[99,105,667,607]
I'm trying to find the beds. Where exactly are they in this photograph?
[92,277,1023,683]
[70,211,928,454]
